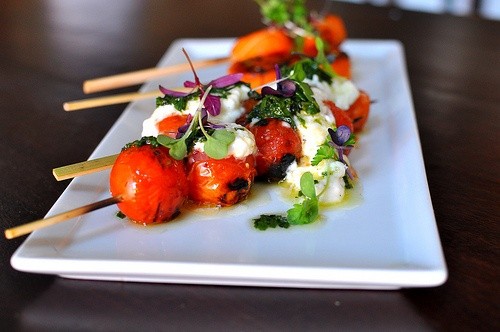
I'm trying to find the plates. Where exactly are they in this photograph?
[9,38,448,290]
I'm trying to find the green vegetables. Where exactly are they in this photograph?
[109,0,371,230]
